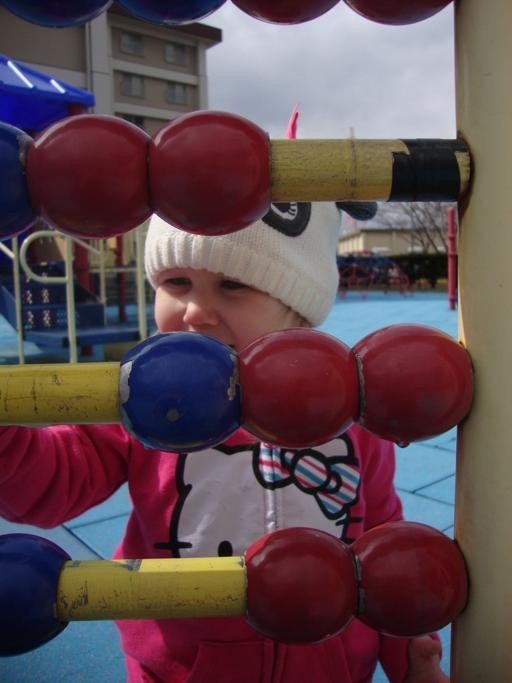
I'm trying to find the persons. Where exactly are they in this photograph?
[1,201,450,683]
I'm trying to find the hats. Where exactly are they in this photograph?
[145,199,377,326]
